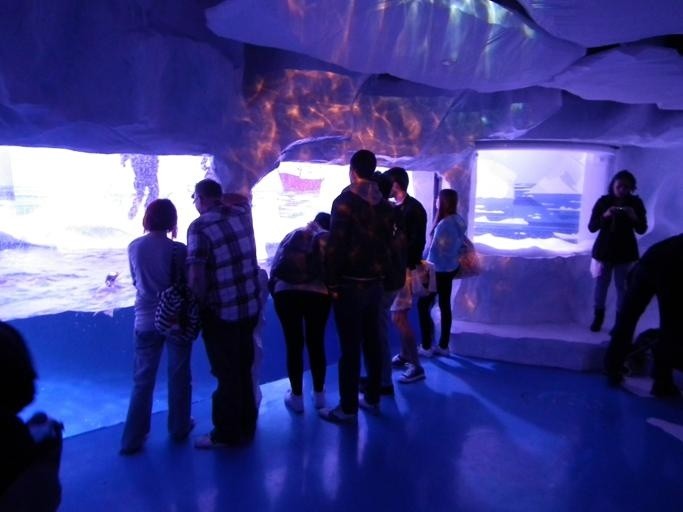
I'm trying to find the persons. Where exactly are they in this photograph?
[417,188,468,358]
[608,232,683,407]
[0,319,65,512]
[268,212,330,414]
[588,169,648,333]
[372,171,394,201]
[184,179,263,452]
[384,167,427,383]
[317,149,394,426]
[119,198,188,457]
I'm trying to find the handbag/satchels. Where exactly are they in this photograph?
[449,233,480,280]
[154,243,202,344]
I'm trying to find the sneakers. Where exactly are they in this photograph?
[650,382,683,403]
[353,396,380,411]
[433,343,450,354]
[591,316,605,331]
[414,344,434,357]
[310,383,324,410]
[357,374,395,392]
[284,388,304,414]
[194,434,218,447]
[118,442,145,455]
[319,407,354,422]
[395,363,426,384]
[390,353,406,366]
[611,372,623,388]
[170,414,194,439]
